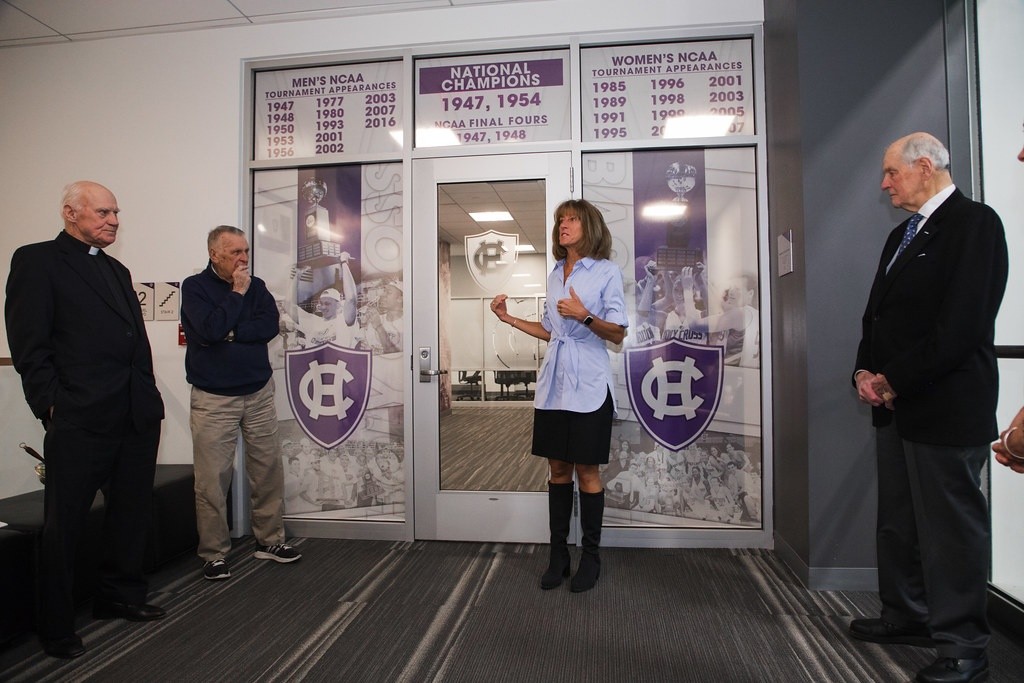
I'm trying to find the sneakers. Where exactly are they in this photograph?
[254,539,302,563]
[203,558,231,578]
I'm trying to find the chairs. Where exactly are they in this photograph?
[451,370,537,402]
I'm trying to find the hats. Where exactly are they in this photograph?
[320,288,339,300]
[301,438,311,446]
[282,439,292,447]
[389,280,403,289]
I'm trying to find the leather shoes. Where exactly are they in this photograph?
[849,618,935,647]
[92,599,166,620]
[916,651,990,682]
[40,624,85,657]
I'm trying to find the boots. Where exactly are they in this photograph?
[541,481,574,588]
[570,488,605,592]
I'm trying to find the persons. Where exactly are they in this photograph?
[179,225,304,579]
[491,198,629,592]
[850,132,1011,682]
[3,180,165,659]
[992,405,1024,474]
[600,251,764,530]
[282,248,411,521]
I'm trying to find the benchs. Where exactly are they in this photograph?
[0,463,234,637]
[0,530,41,651]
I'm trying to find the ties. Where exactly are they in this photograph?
[898,213,924,257]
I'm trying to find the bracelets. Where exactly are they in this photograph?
[1004,427,1023,460]
[512,317,517,327]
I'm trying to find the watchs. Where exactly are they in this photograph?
[581,312,594,326]
